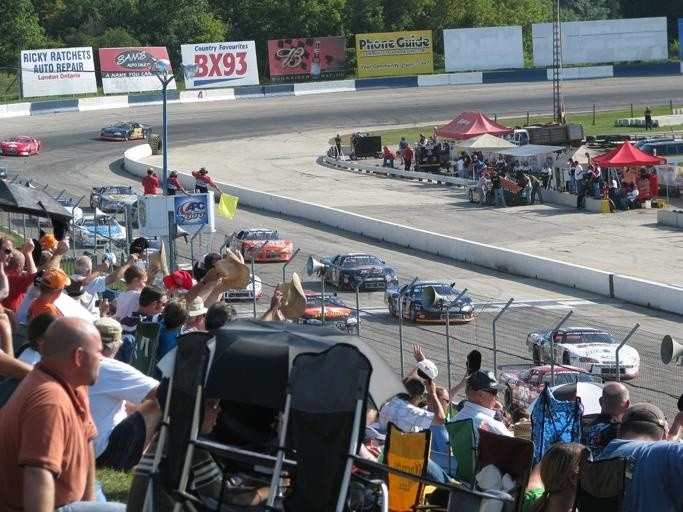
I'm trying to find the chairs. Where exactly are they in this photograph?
[111,313,637,510]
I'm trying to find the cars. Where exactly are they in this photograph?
[174,263,199,294]
[633,137,674,149]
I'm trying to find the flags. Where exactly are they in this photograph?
[216,193,239,220]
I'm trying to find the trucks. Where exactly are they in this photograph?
[510,122,584,146]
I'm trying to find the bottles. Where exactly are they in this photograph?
[102,298,109,313]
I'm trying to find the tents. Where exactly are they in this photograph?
[434,111,668,181]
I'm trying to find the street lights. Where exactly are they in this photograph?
[145,58,201,299]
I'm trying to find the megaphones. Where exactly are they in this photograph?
[95,207,110,219]
[307,256,330,274]
[661,335,683,364]
[421,286,448,306]
[175,224,190,237]
[25,182,36,188]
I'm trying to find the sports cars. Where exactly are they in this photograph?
[105,233,162,269]
[223,274,263,302]
[384,281,475,323]
[0,135,42,157]
[29,183,144,247]
[469,173,525,206]
[224,228,295,263]
[275,289,361,335]
[101,121,153,142]
[527,326,639,382]
[317,252,401,292]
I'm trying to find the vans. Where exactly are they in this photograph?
[639,140,683,177]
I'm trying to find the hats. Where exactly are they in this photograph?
[417,359,439,381]
[34,268,85,297]
[621,402,669,429]
[130,238,193,290]
[187,296,209,317]
[277,271,307,321]
[38,219,69,249]
[466,370,507,390]
[215,247,249,289]
[94,316,122,343]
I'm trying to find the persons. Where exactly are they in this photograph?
[167,170,189,196]
[644,105,652,131]
[453,151,658,213]
[143,170,158,195]
[191,168,223,195]
[0,232,290,512]
[343,345,683,512]
[334,134,342,158]
[351,132,370,159]
[399,133,450,172]
[381,145,396,166]
